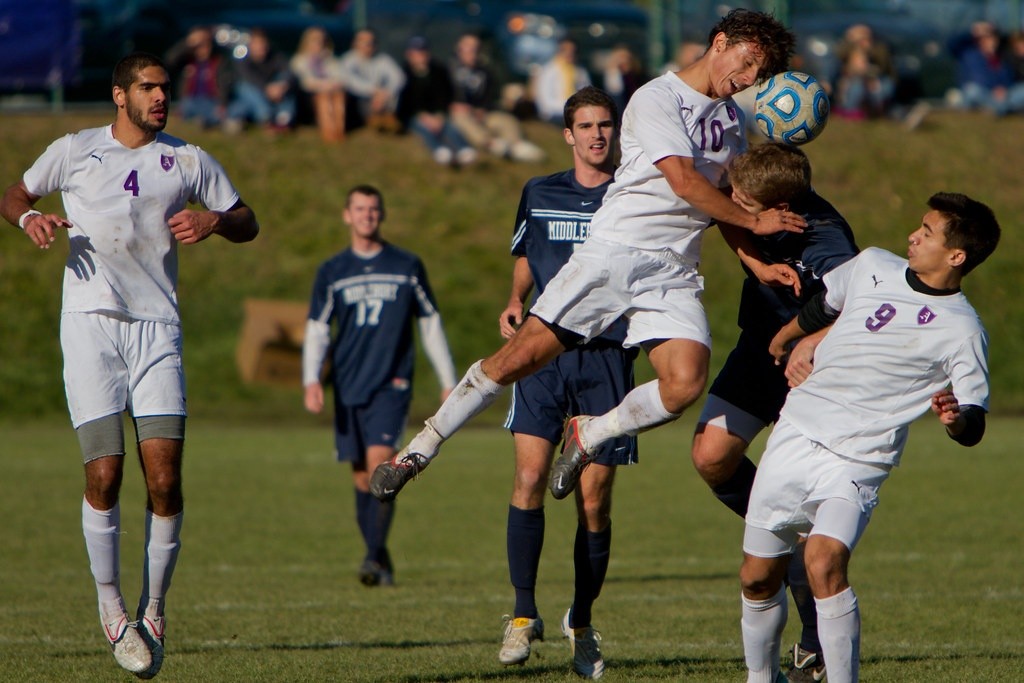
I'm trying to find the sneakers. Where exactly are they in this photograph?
[499,612,544,665]
[781,644,826,683]
[562,607,604,678]
[131,607,166,680]
[358,557,394,584]
[100,606,154,672]
[371,444,430,498]
[549,415,598,499]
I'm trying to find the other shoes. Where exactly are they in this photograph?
[488,137,509,157]
[507,140,547,162]
[433,148,476,163]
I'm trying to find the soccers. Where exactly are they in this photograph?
[755,71,831,146]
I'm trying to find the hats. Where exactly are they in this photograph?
[404,31,431,51]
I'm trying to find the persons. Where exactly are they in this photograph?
[738,193,1001,683]
[0,52,261,680]
[367,7,808,501]
[499,86,640,678]
[690,139,860,683]
[174,9,1024,170]
[302,184,459,587]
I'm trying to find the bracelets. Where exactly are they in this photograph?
[19,210,42,228]
